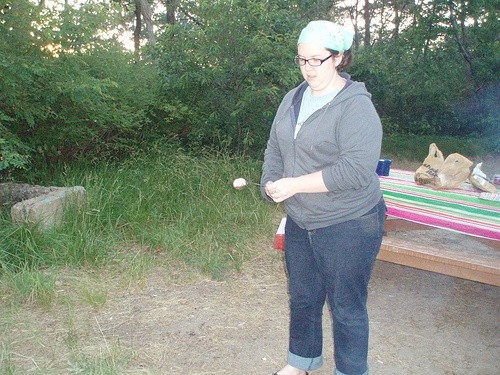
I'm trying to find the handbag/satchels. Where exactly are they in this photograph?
[430,153,474,190]
[469,161,497,194]
[414,142,444,185]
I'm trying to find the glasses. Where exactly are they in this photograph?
[293,52,336,67]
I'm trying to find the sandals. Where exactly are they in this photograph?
[271,368,309,375]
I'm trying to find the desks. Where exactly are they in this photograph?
[375,168,500,286]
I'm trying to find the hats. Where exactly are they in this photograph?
[297,19,354,52]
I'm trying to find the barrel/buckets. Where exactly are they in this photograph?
[376,159,392,176]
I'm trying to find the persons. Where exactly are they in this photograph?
[256,18,387,375]
